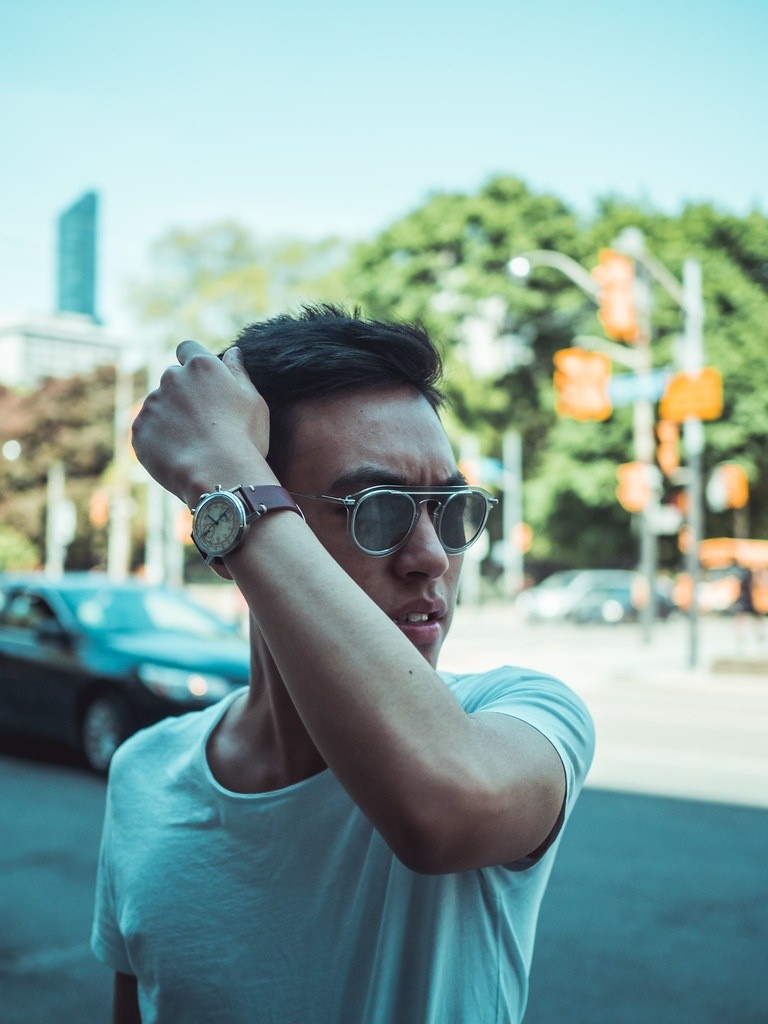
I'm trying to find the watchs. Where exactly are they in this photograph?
[190,484,297,566]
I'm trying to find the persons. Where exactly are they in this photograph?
[91,302,597,1024]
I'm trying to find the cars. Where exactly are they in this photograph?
[0,570,252,778]
[512,568,674,630]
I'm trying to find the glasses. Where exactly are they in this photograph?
[281,483,499,558]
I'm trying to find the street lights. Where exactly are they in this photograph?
[612,224,706,670]
[508,246,658,634]
